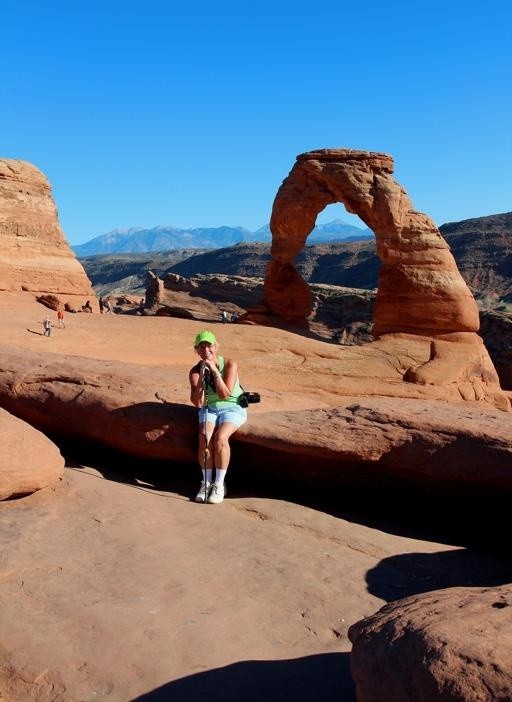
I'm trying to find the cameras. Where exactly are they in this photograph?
[237,391,260,408]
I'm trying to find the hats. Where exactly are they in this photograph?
[194,331,218,346]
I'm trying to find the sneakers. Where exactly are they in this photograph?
[194,479,228,504]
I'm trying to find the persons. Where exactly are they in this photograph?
[86,300,93,313]
[103,295,111,314]
[222,309,229,324]
[231,312,237,322]
[190,330,248,505]
[99,297,104,314]
[43,316,52,337]
[57,309,66,328]
[139,299,146,307]
